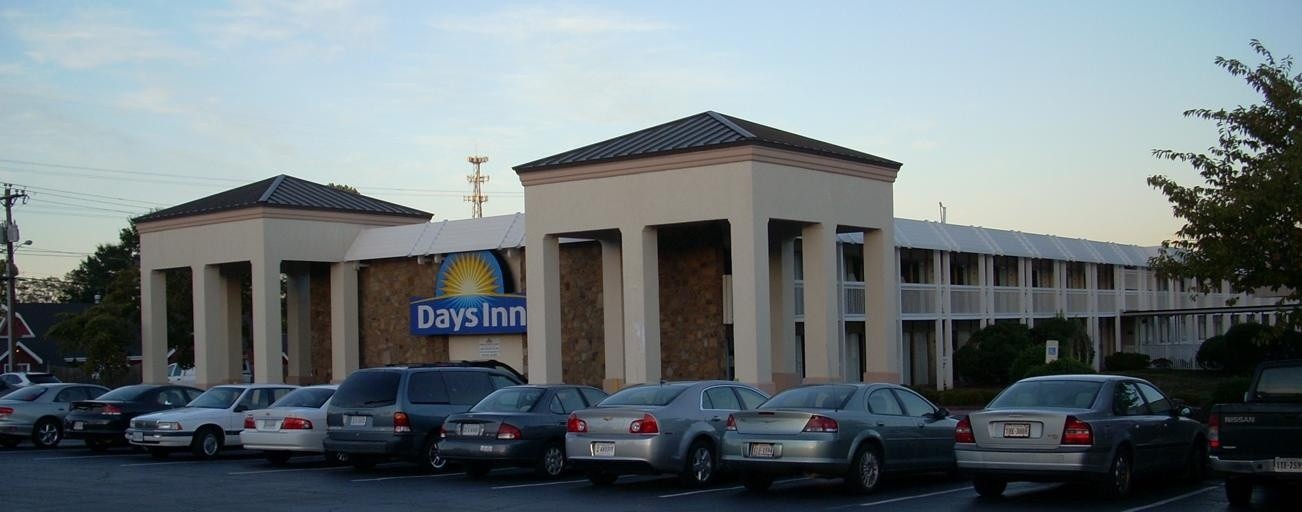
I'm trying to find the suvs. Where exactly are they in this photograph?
[0,372,63,388]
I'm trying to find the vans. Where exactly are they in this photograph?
[322,360,529,475]
[167,354,251,386]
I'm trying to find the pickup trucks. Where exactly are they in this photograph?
[1206,357,1301,504]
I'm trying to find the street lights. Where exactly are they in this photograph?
[5,239,33,372]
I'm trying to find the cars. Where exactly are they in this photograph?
[565,379,771,488]
[239,383,355,467]
[718,381,971,495]
[124,384,303,462]
[437,383,612,482]
[1,376,25,447]
[0,382,112,448]
[953,374,1208,504]
[64,384,224,453]
